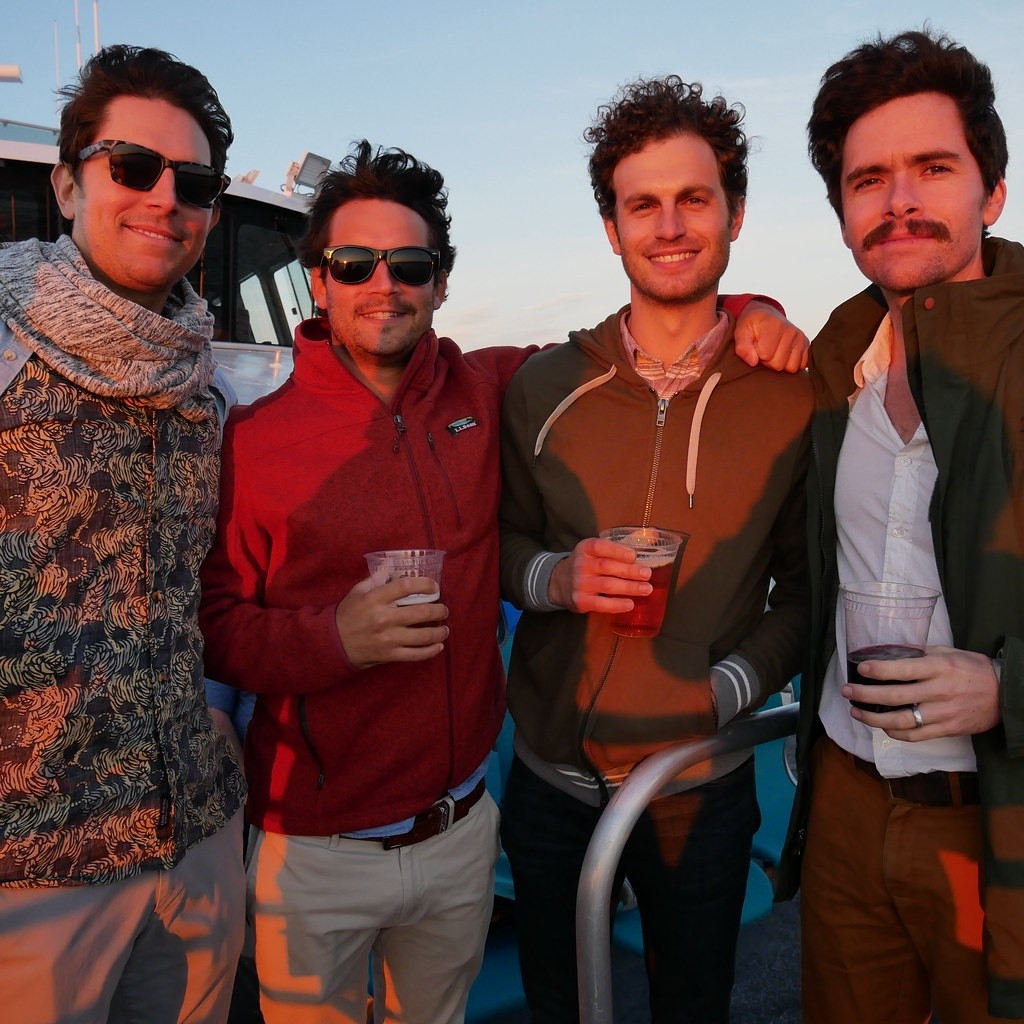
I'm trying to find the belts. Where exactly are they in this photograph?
[837,745,980,806]
[328,774,485,850]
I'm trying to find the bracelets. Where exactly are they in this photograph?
[993,657,1001,687]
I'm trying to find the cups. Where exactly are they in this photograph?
[363,550,448,648]
[598,526,683,638]
[838,582,942,713]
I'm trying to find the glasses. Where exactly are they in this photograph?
[321,246,440,285]
[70,139,231,209]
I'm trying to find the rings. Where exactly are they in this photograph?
[913,707,923,727]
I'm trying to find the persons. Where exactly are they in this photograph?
[201,141,806,1024]
[498,77,813,1023]
[2,48,245,1024]
[775,33,1024,1024]
